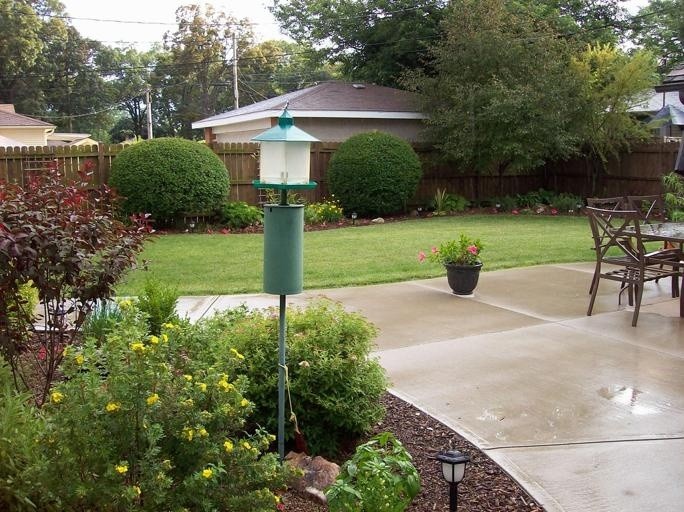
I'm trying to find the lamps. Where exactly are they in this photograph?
[351,211,357,225]
[435,437,470,512]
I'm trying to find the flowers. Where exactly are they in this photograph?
[417,232,484,266]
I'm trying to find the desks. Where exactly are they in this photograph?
[607,222,684,317]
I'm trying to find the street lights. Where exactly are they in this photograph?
[248,97,318,456]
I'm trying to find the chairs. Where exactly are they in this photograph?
[581,195,676,308]
[620,194,681,289]
[584,206,684,326]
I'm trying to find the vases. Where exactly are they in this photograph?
[442,260,483,295]
